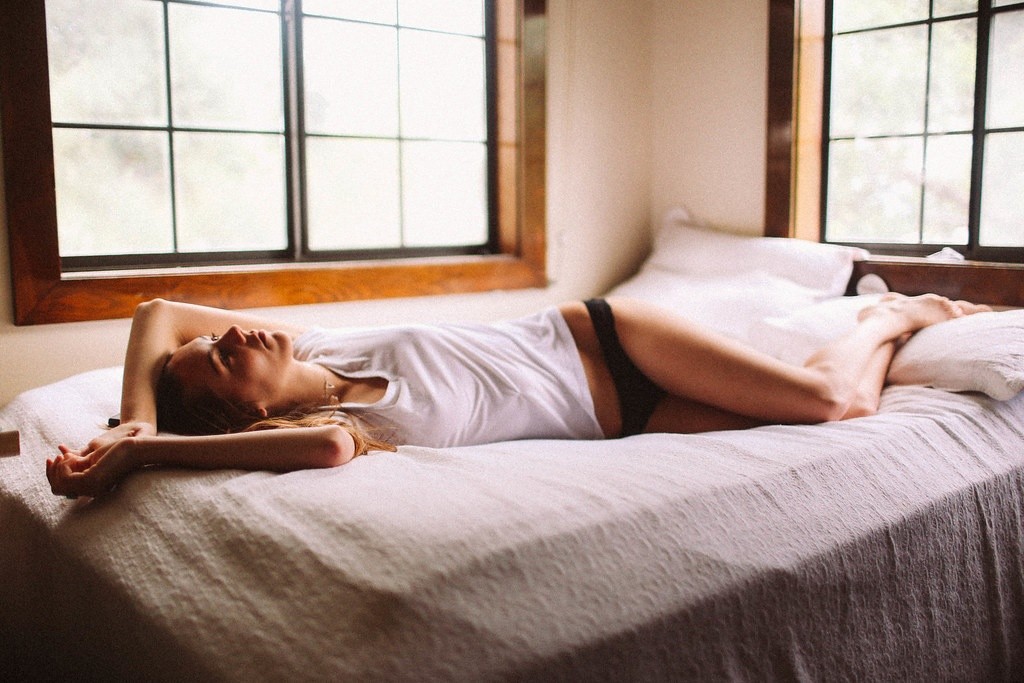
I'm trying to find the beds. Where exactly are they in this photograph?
[0,256,1024,683]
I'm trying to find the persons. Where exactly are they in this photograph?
[45,292,994,496]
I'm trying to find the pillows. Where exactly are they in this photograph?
[602,207,863,370]
[762,290,1024,403]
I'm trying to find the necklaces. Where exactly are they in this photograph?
[325,368,336,404]
